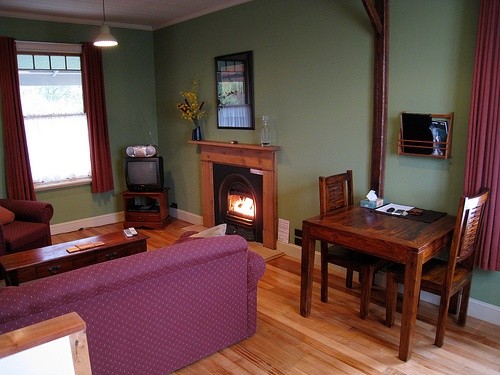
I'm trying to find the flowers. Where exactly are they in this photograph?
[177,78,206,123]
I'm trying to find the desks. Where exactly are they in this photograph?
[298,202,459,364]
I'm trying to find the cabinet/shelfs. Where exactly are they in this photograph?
[120,190,170,231]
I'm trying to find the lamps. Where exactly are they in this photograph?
[93,0,119,48]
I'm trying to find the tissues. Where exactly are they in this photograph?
[360,190,384,209]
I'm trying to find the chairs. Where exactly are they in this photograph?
[386,188,490,347]
[319,169,383,320]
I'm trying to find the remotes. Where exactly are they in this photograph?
[123,229,133,237]
[129,227,138,235]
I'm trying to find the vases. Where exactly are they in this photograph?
[191,126,202,142]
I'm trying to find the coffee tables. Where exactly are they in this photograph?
[0,230,148,287]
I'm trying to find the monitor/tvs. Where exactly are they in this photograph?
[125,156,165,193]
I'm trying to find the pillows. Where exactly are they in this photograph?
[0,205,15,224]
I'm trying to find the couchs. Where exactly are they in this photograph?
[0,227,266,375]
[0,197,55,255]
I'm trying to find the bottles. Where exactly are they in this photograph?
[261,114,270,146]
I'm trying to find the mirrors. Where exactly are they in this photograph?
[213,50,255,130]
